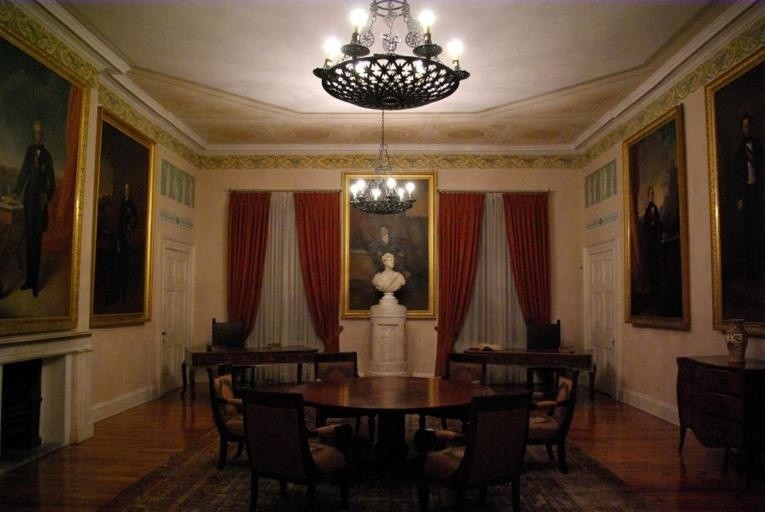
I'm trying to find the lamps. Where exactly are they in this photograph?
[309,1,471,218]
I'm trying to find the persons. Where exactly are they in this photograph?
[115,183,140,309]
[364,226,420,253]
[733,114,764,323]
[642,185,667,316]
[370,253,408,296]
[10,119,54,299]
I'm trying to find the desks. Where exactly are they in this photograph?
[444,345,596,402]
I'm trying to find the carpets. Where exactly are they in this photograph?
[95,409,656,512]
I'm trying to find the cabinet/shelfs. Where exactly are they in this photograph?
[673,355,764,488]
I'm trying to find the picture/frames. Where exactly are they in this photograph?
[339,163,438,320]
